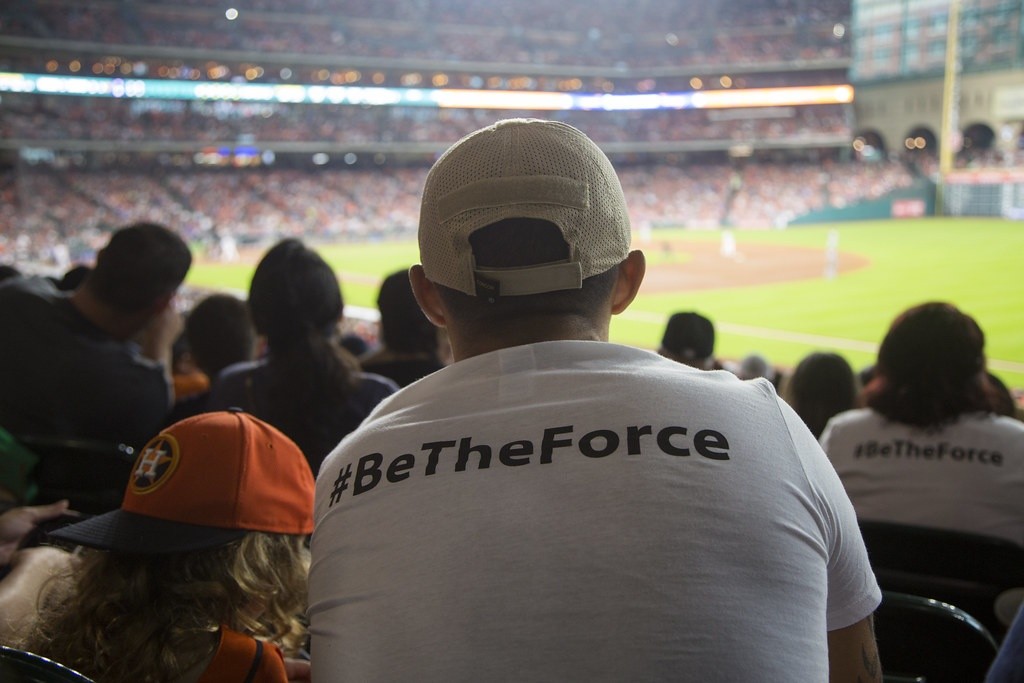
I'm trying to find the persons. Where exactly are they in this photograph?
[0,221,455,683]
[309,118,883,683]
[658,302,1024,683]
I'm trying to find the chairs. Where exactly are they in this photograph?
[852,518,1024,683]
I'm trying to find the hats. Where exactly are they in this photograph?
[417,118,631,298]
[120,408,317,533]
